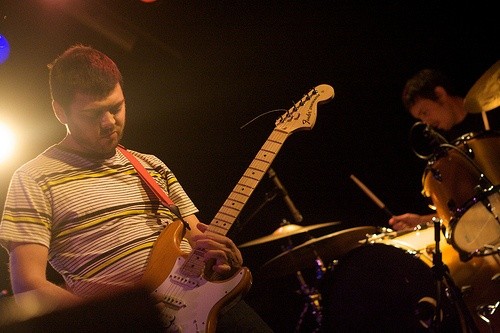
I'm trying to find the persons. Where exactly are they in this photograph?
[0,42,273,333]
[386,64,500,237]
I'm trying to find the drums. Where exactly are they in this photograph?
[297,221,499,333]
[421,128,500,263]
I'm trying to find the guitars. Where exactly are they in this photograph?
[137,84,335,332]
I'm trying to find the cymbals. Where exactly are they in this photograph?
[235,220,343,250]
[257,226,380,278]
[463,59,500,114]
